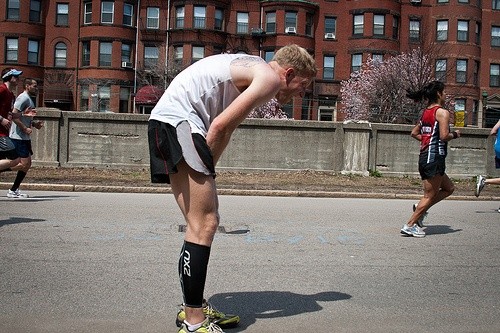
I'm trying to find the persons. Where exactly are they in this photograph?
[400,81,460,237]
[475,119,500,213]
[0,68,43,198]
[148,44,318,333]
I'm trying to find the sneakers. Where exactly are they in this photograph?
[400,224,426,237]
[413,203,428,228]
[497,208,500,212]
[177,316,226,333]
[7,188,30,198]
[474,174,486,197]
[175,299,240,329]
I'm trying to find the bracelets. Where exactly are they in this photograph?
[451,132,457,139]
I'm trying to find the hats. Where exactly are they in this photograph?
[2,68,23,79]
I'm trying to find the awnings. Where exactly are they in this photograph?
[135,85,160,105]
